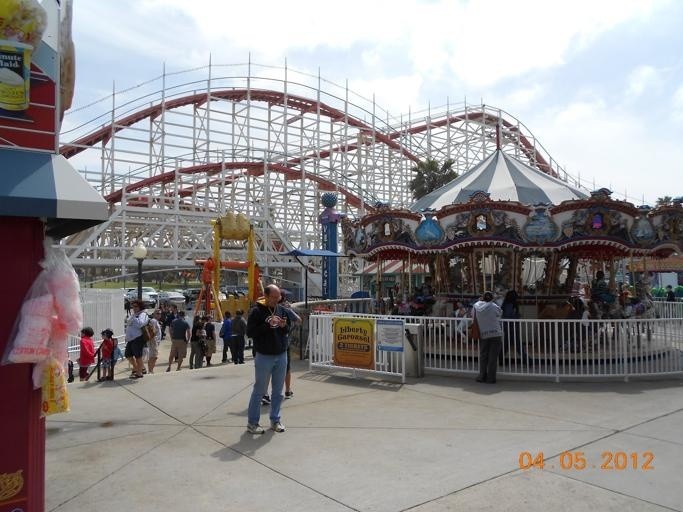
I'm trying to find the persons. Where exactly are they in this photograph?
[661,282,680,302]
[368,269,653,386]
[246,284,290,435]
[78,298,248,382]
[257,292,302,406]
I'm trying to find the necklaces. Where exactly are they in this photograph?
[265,304,277,315]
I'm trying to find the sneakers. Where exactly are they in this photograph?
[284,391,293,399]
[246,422,264,434]
[262,394,271,404]
[270,419,285,432]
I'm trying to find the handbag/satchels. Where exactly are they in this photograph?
[142,322,157,342]
[471,307,479,339]
[196,329,207,337]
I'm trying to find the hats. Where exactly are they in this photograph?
[177,311,185,316]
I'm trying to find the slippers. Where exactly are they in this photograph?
[129,374,142,379]
